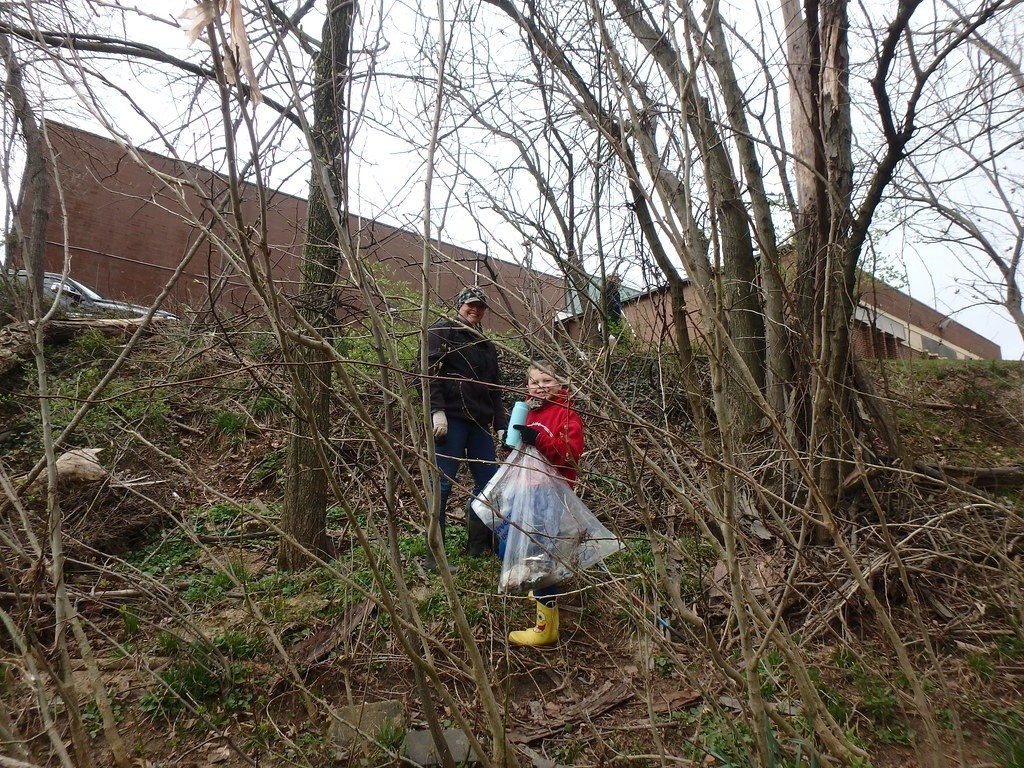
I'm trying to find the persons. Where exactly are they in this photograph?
[412,284,512,575]
[467,358,628,648]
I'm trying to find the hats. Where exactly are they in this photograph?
[458,285,486,309]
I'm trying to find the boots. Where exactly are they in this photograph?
[467,519,492,560]
[528,591,537,602]
[425,523,457,575]
[509,600,560,646]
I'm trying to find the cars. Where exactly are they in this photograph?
[4,267,186,322]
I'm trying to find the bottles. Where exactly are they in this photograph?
[505,402,530,448]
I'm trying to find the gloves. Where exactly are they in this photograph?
[432,410,447,436]
[501,432,514,451]
[497,430,505,442]
[513,425,540,447]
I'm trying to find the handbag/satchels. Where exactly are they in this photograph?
[471,440,626,598]
